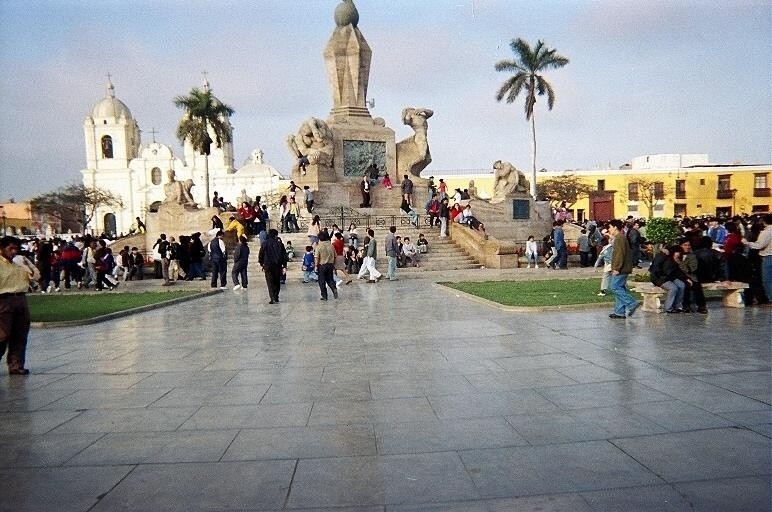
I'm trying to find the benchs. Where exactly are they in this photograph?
[631,281,752,312]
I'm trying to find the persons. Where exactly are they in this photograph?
[297,149,308,176]
[489,159,524,193]
[401,106,434,153]
[288,117,335,166]
[364,164,379,179]
[526,201,771,319]
[162,170,183,203]
[1,237,41,375]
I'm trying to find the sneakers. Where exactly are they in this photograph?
[39,275,248,294]
[667,307,709,314]
[267,223,446,304]
[527,261,560,269]
[9,368,29,375]
[597,290,642,318]
